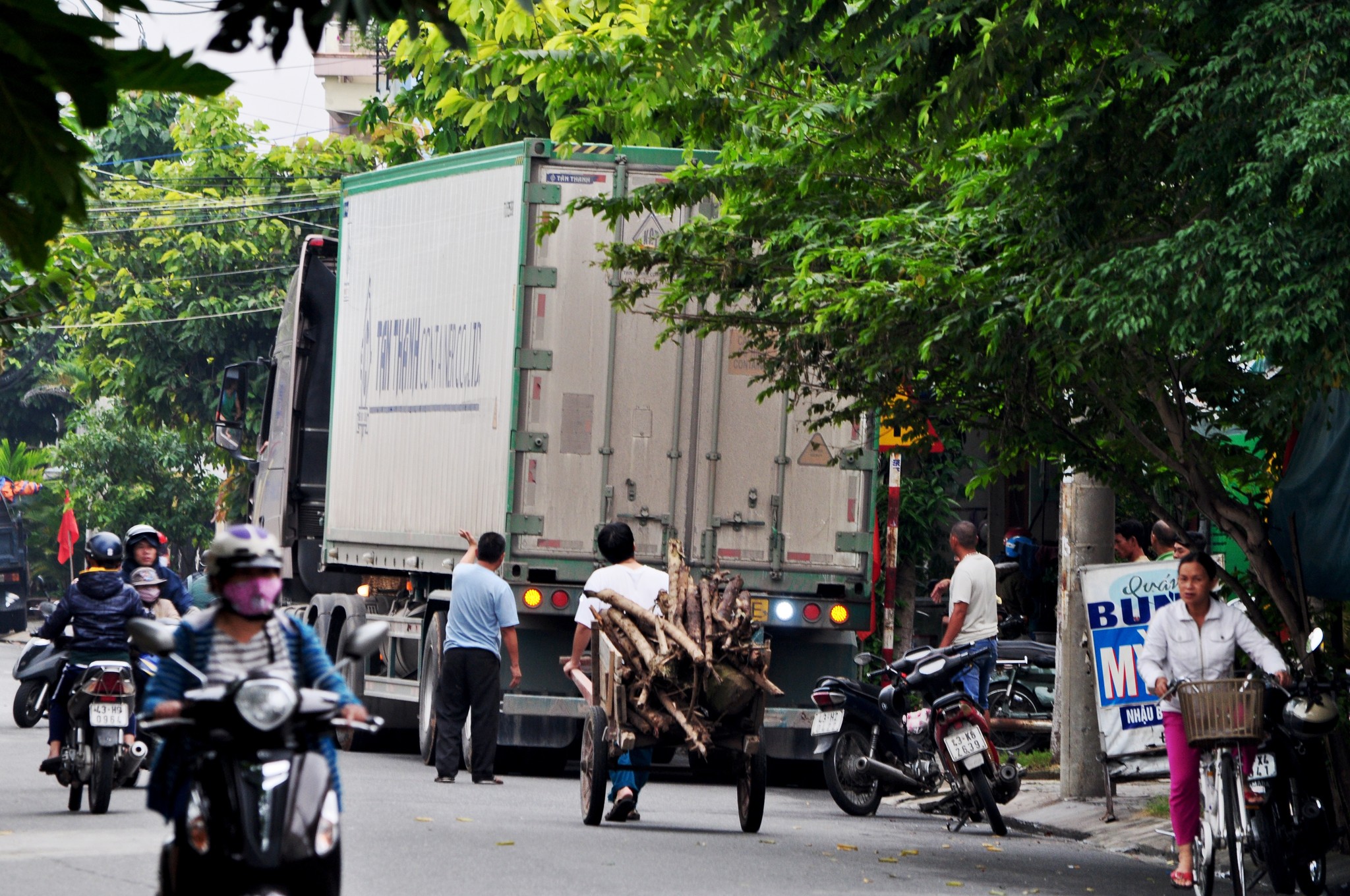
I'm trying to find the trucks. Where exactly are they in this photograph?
[211,136,886,779]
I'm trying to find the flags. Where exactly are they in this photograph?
[57,501,80,563]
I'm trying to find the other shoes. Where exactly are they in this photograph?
[474,775,504,784]
[39,757,62,772]
[435,776,455,782]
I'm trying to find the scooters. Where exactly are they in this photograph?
[1231,624,1350,896]
[122,617,394,896]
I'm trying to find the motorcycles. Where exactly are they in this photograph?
[13,572,148,816]
[810,521,1055,836]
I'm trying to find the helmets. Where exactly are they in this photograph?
[1002,527,1030,546]
[208,524,283,585]
[122,524,160,557]
[84,532,123,568]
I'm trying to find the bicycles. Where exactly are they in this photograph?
[1153,665,1296,896]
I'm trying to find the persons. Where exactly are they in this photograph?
[1173,531,1208,561]
[1114,528,1149,562]
[562,521,670,822]
[121,526,218,721]
[930,520,999,730]
[37,531,146,773]
[1134,552,1292,888]
[1150,521,1181,562]
[433,529,522,784]
[141,523,369,812]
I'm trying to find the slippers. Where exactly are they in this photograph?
[605,798,634,822]
[1244,792,1266,807]
[628,810,641,820]
[1170,871,1193,890]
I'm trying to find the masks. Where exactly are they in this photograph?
[222,573,283,615]
[137,587,161,603]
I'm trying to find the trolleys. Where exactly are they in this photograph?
[575,632,768,835]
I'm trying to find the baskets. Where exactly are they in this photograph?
[1177,679,1266,750]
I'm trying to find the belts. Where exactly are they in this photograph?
[986,635,996,641]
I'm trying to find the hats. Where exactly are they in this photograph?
[130,566,168,585]
